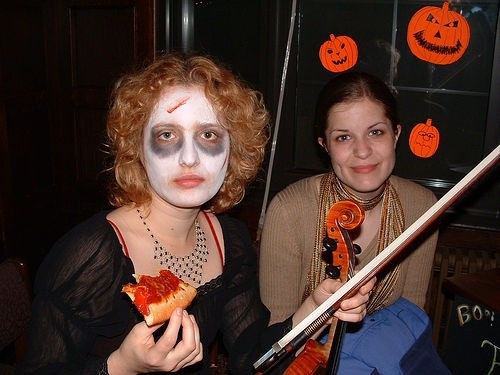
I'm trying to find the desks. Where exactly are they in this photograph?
[441,267,500,375]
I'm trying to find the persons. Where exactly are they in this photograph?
[23,56,376,374]
[252,73,444,375]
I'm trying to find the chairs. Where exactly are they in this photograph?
[0,254,35,375]
[423,223,500,375]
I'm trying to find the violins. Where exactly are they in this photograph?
[279,199,368,375]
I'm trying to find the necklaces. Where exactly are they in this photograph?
[135,204,212,288]
[302,170,405,318]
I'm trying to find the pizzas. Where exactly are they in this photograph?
[121,270,197,327]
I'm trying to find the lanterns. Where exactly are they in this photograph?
[319,32,360,73]
[408,118,440,158]
[406,1,472,67]
